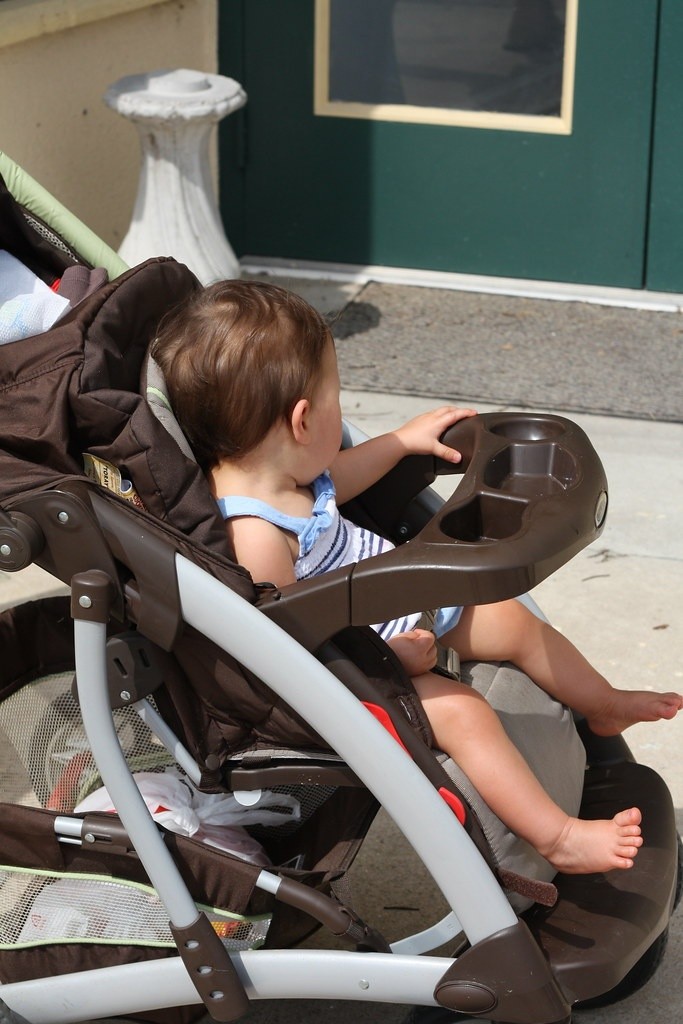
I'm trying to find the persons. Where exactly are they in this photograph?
[152,279,683,873]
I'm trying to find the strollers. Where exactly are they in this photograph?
[0,150,683,1023]
